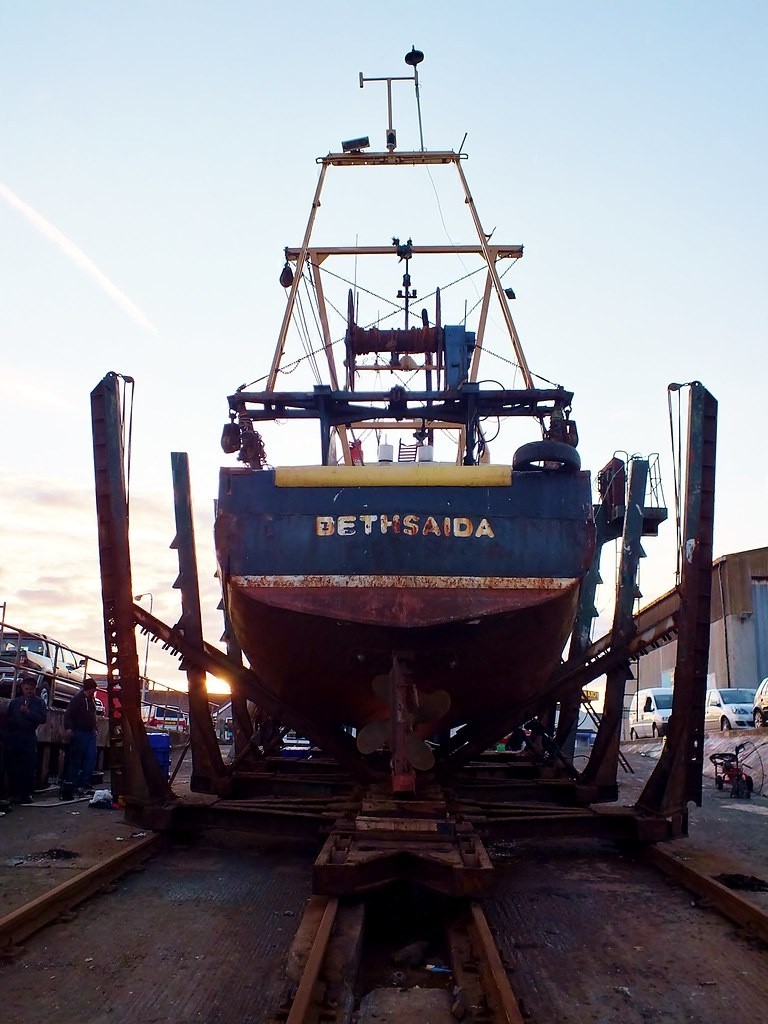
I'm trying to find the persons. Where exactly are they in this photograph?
[4,679,47,803]
[61,679,98,790]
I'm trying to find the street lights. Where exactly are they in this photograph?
[134,591,154,701]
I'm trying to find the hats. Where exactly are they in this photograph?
[84,679,97,691]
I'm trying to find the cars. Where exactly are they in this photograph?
[94,698,106,714]
[704,688,756,732]
[496,729,531,749]
[752,677,768,733]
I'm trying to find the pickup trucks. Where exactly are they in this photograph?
[0,630,98,710]
[275,737,313,760]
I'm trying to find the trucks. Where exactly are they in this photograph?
[555,728,598,746]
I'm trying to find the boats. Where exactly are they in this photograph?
[207,41,667,792]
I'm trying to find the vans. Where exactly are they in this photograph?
[628,688,673,739]
[141,704,189,733]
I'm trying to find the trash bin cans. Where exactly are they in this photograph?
[146,732,171,793]
